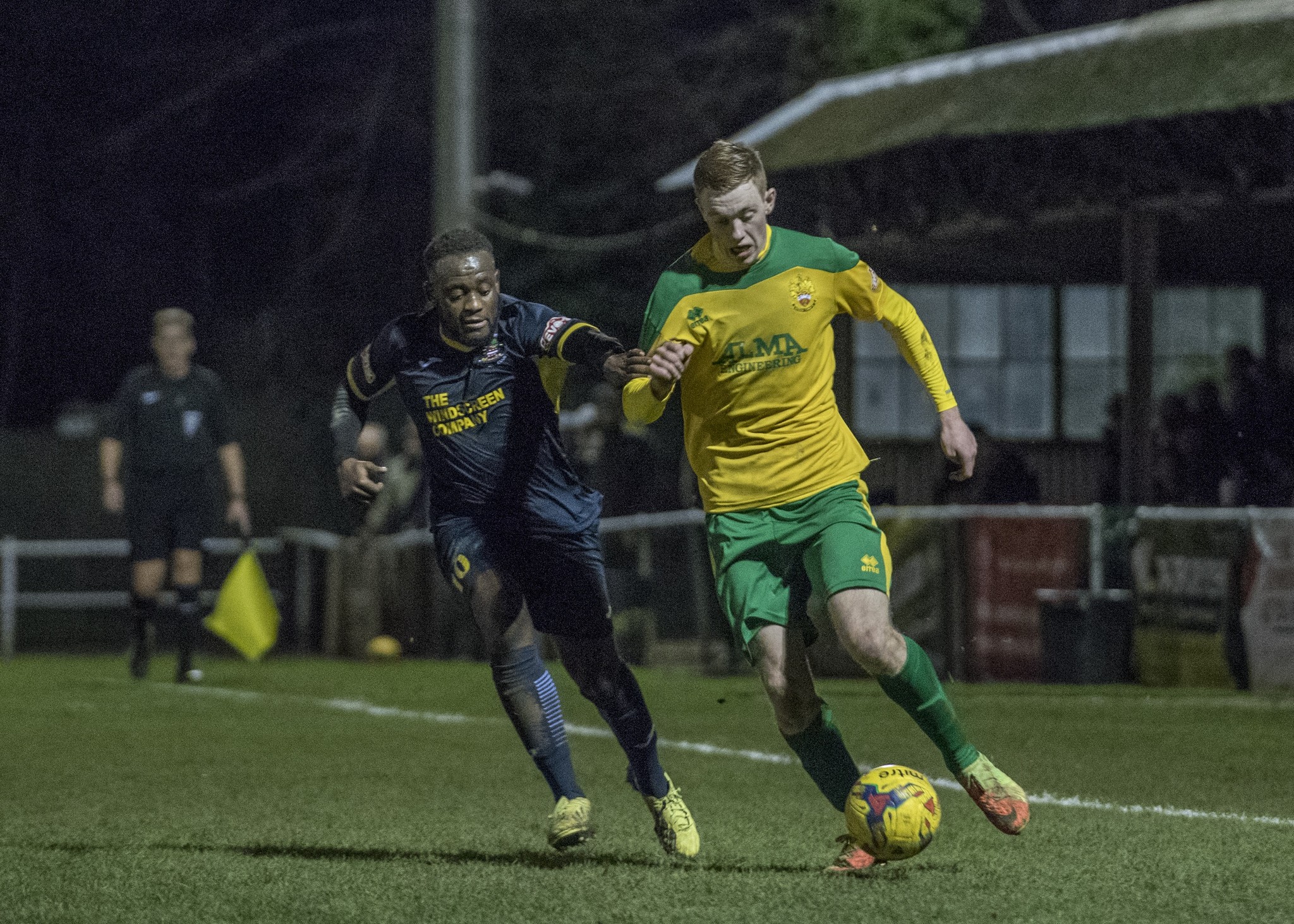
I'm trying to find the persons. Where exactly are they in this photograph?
[98,306,253,687]
[617,133,1032,878]
[340,338,1294,696]
[330,225,706,862]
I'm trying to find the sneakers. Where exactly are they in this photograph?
[823,838,887,874]
[546,794,598,852]
[625,759,701,860]
[954,750,1030,835]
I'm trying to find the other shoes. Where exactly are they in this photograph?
[175,665,204,685]
[130,623,159,678]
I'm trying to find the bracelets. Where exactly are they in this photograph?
[221,487,247,504]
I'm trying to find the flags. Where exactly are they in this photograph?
[200,539,283,666]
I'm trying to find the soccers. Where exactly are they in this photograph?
[843,763,942,863]
